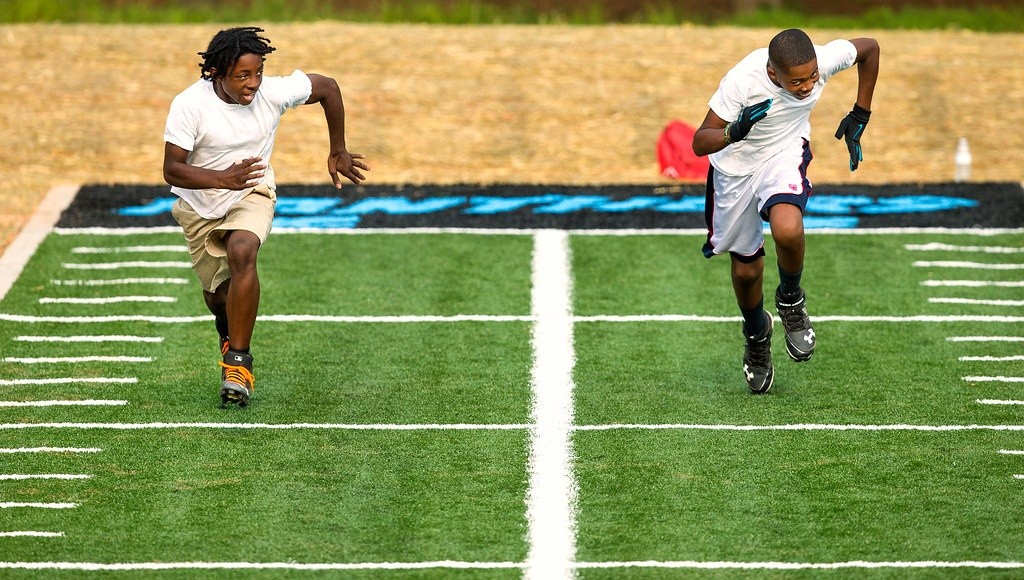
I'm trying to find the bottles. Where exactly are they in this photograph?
[953,138,971,181]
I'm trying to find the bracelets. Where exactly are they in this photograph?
[724,122,734,144]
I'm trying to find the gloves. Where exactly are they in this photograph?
[835,103,872,171]
[730,98,774,142]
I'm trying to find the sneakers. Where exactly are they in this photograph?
[774,284,816,362]
[219,334,230,357]
[742,309,774,395]
[218,350,255,409]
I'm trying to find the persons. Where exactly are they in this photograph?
[162,27,371,408]
[692,28,880,394]
